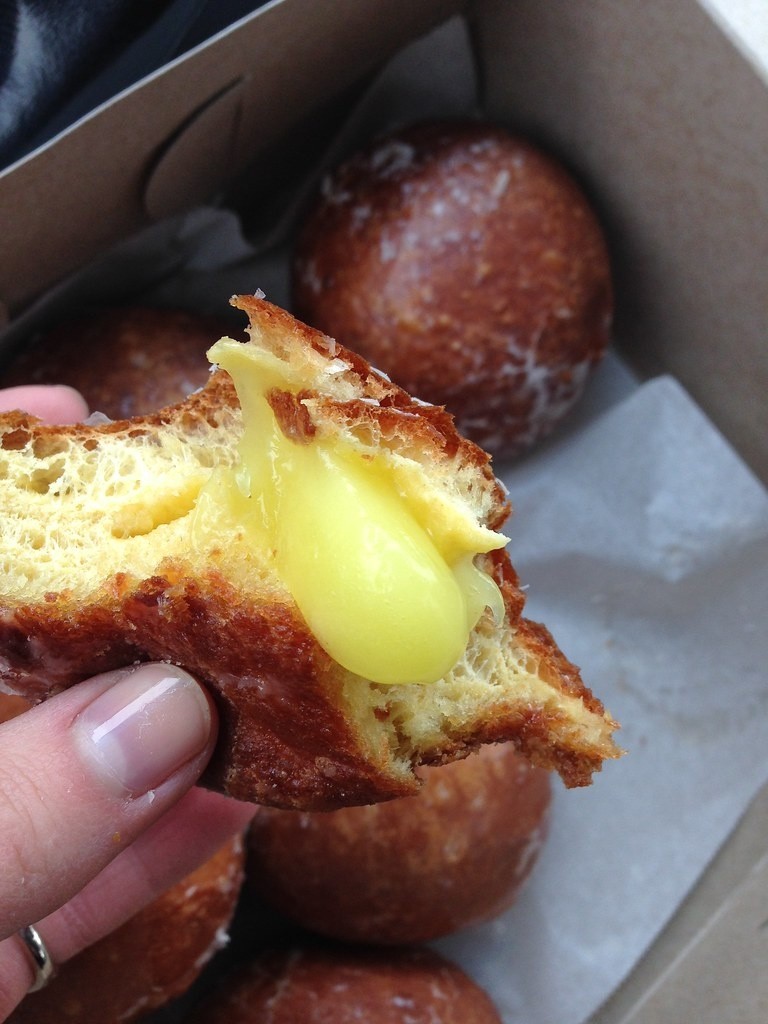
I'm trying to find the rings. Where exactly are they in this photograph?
[18,924,54,993]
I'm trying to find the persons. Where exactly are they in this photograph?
[0,383,267,1024]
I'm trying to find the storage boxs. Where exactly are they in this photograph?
[3,1,768,1022]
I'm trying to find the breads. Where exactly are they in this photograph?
[0,122,626,1024]
